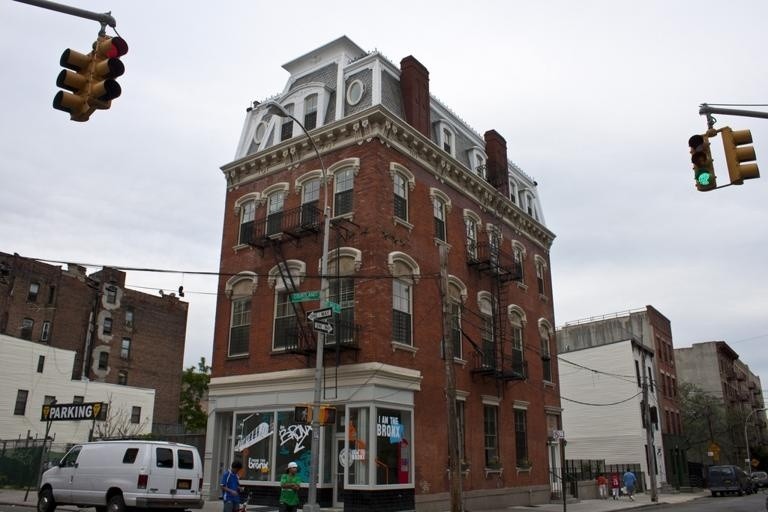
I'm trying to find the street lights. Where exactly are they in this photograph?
[744,408,768,474]
[266,100,333,511]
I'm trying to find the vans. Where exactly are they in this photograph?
[37,440,205,511]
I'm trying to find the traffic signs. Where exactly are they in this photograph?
[306,307,336,336]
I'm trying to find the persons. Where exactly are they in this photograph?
[219,460,243,512]
[598,473,609,500]
[279,461,301,512]
[610,473,620,500]
[623,468,637,501]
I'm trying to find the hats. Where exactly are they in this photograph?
[288,462,297,469]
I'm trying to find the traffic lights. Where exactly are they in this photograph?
[53,26,128,121]
[293,403,338,426]
[688,127,760,190]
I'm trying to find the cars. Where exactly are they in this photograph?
[707,465,767,496]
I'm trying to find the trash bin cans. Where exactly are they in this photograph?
[571,481,578,498]
